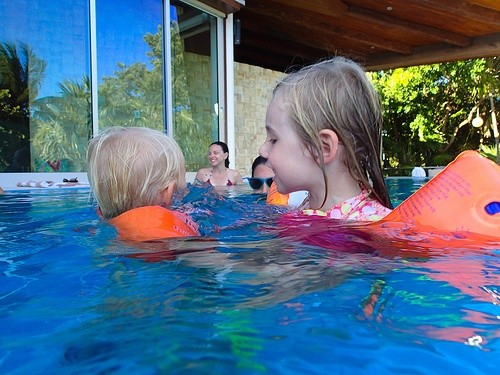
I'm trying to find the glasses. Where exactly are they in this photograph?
[247,176,273,190]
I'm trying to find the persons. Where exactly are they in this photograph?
[47,181,54,186]
[85,127,200,240]
[258,56,394,225]
[249,154,276,194]
[62,177,79,183]
[192,141,245,186]
